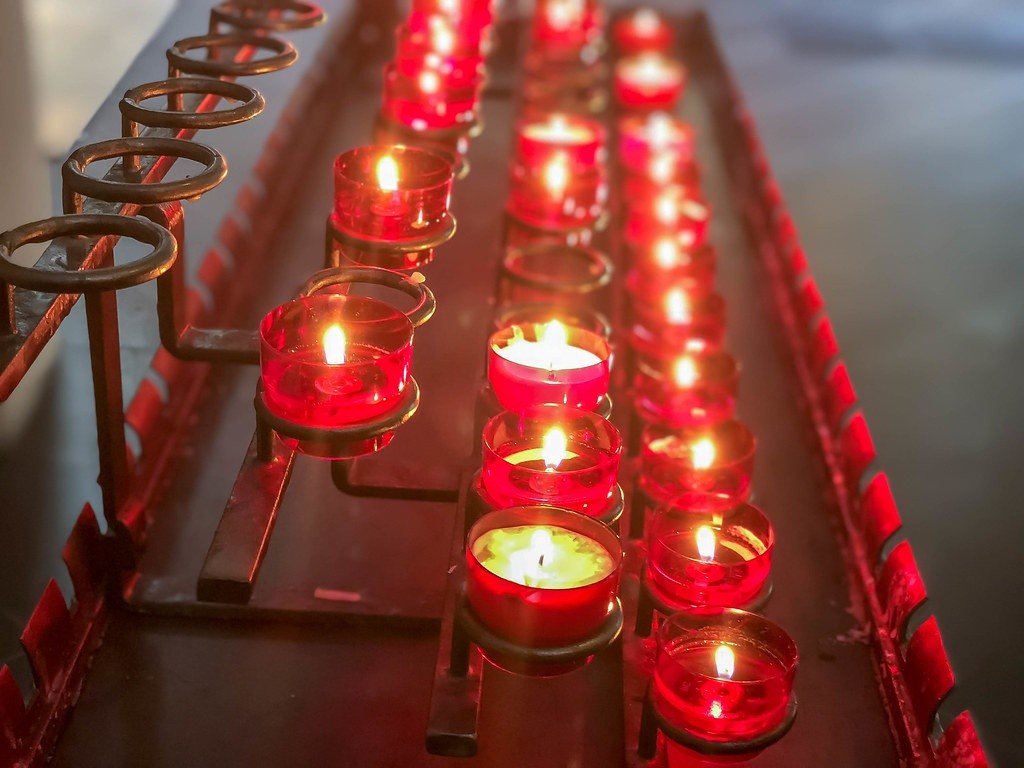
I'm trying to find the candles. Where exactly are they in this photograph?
[331,144,456,241]
[507,0,606,229]
[479,404,624,516]
[614,2,801,744]
[464,502,621,649]
[489,315,621,419]
[259,295,414,429]
[380,0,492,129]
